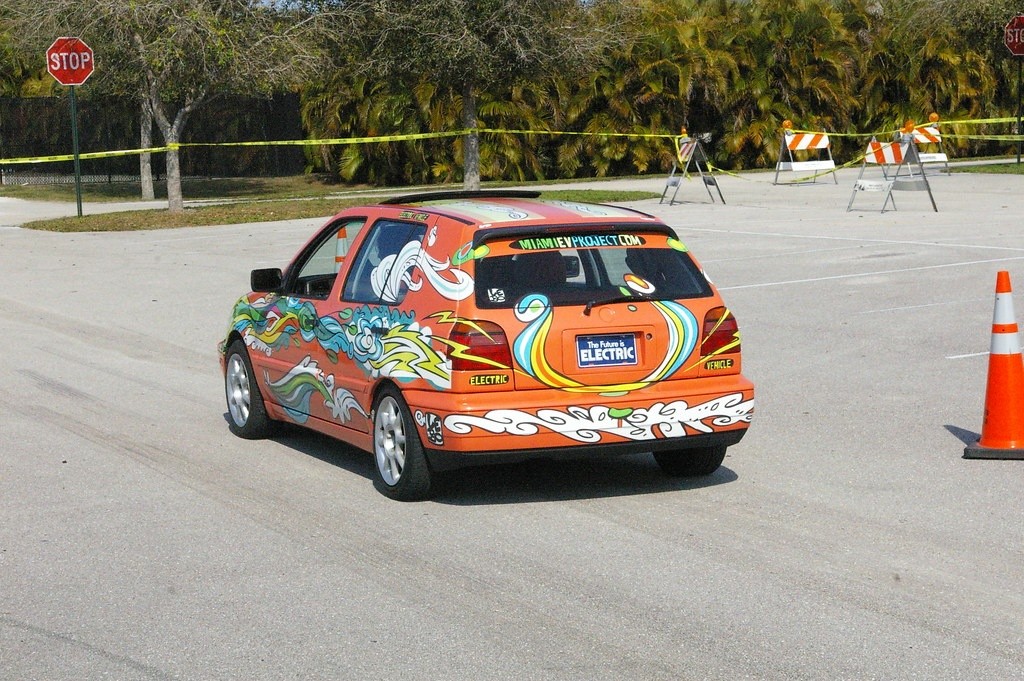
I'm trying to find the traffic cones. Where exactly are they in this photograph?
[960,270,1024,461]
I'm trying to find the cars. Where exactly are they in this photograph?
[216,187,756,501]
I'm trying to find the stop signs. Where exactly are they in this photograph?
[45,36,94,86]
[1004,15,1024,55]
